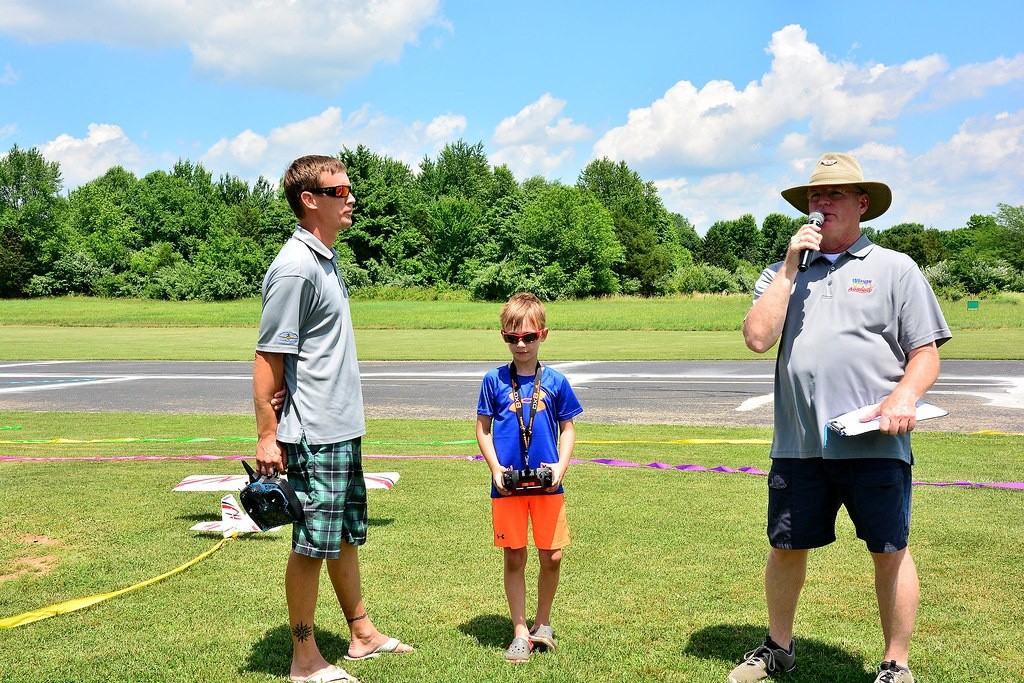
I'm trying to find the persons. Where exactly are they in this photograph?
[475,293,584,663]
[727,152,954,683]
[252,155,418,683]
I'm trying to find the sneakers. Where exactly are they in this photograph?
[728,633,796,683]
[874,660,914,683]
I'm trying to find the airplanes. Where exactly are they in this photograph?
[171,468,400,540]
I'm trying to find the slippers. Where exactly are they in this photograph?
[288,664,359,683]
[505,638,534,663]
[343,635,414,661]
[529,625,557,650]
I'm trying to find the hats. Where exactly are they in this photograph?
[780,153,892,223]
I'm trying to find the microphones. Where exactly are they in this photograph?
[797,210,825,273]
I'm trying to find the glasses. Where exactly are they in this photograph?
[502,329,543,343]
[303,185,354,199]
[804,188,862,202]
